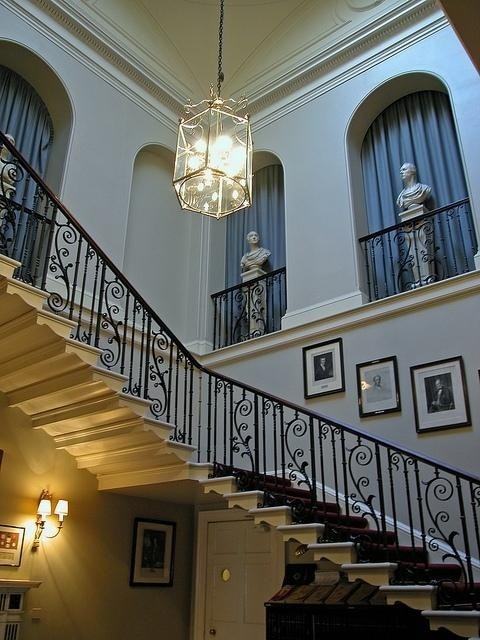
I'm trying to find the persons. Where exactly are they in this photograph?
[240,230,271,269]
[396,162,431,209]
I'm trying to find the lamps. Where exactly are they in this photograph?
[33,493,70,548]
[172,0,254,221]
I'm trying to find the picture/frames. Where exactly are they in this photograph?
[410,355,472,434]
[355,355,401,418]
[129,516,177,589]
[302,337,345,399]
[0,524,25,567]
